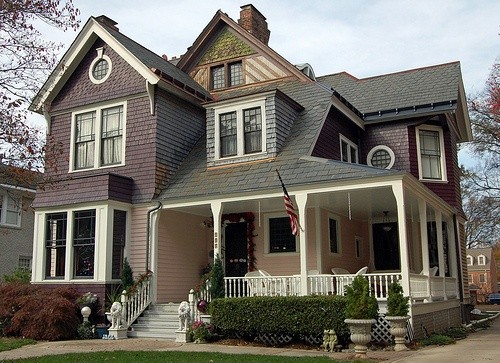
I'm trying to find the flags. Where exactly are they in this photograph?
[279,181,299,238]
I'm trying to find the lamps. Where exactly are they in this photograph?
[381,210,393,232]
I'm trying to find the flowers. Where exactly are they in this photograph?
[191,319,214,338]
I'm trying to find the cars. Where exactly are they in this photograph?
[485,293,500,305]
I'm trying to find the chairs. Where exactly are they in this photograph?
[308,265,369,294]
[245,270,281,297]
[418,266,438,277]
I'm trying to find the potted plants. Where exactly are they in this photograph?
[105,289,122,324]
[383,282,412,351]
[342,275,380,359]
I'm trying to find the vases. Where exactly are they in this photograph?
[193,332,210,345]
